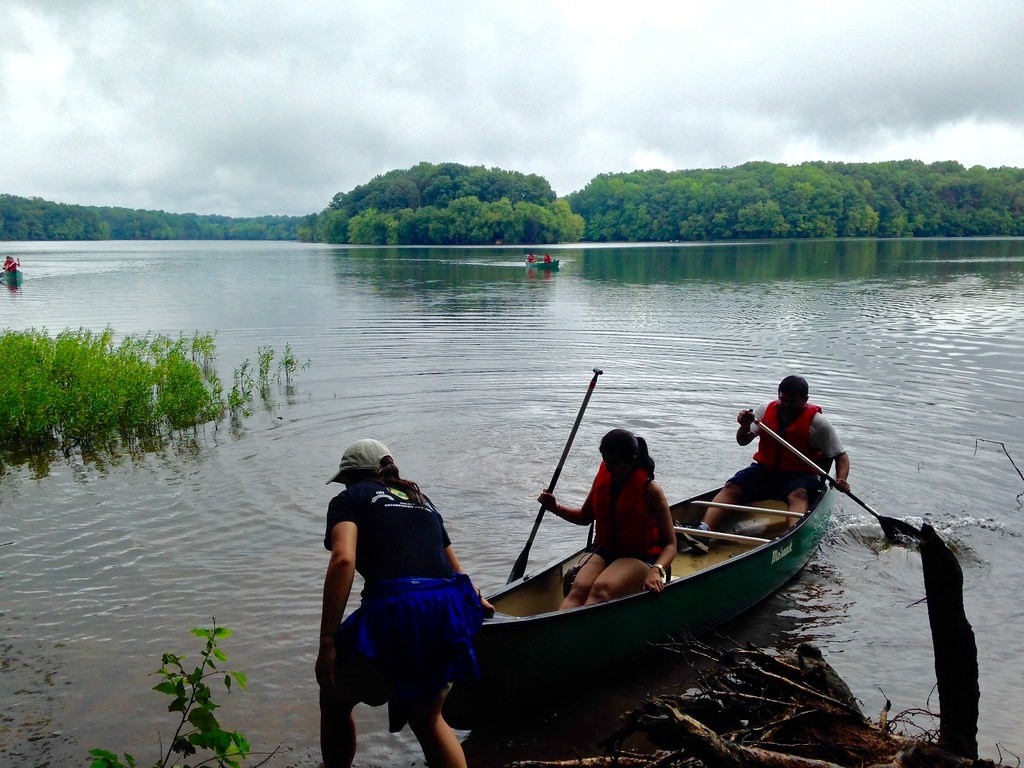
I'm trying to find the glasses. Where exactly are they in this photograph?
[778,396,806,406]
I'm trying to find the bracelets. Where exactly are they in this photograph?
[320,631,337,637]
[475,585,483,600]
[652,563,666,578]
[555,505,561,516]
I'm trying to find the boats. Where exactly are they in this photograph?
[440,479,834,731]
[0,269,22,280]
[524,259,560,270]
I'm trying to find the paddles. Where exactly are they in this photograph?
[0,259,18,278]
[504,367,604,584]
[748,408,921,542]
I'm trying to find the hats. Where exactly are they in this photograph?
[324,439,394,485]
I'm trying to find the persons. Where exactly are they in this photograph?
[543,251,552,263]
[674,375,849,554]
[314,442,495,768]
[3,256,20,271]
[528,252,536,263]
[537,430,678,612]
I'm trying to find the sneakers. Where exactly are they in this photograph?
[673,519,710,553]
